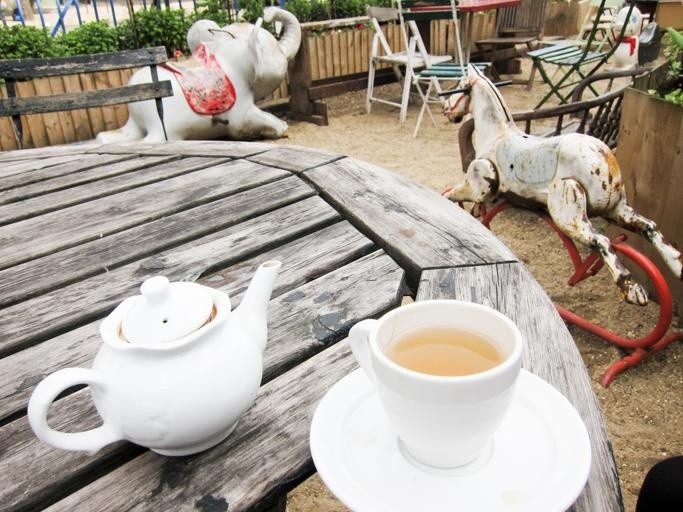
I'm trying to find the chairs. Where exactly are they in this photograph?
[457,0,674,177]
[0,46,174,149]
[364,0,493,140]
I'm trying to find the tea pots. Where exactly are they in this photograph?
[27,255,283,458]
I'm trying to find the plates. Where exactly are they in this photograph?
[307,358,593,512]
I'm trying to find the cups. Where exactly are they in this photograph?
[345,297,527,472]
